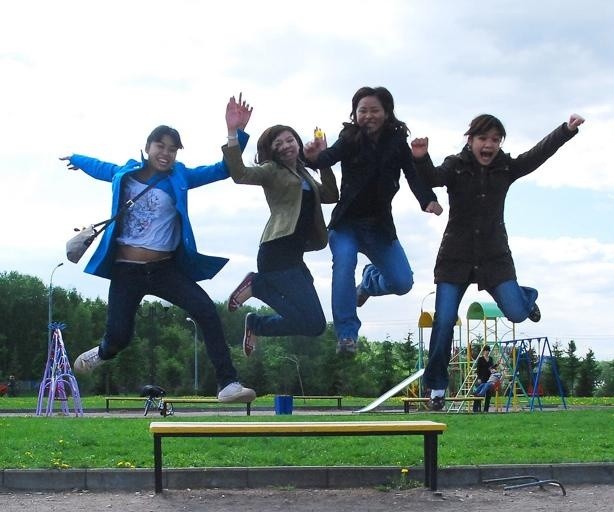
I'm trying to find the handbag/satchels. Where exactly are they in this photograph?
[67,223,99,263]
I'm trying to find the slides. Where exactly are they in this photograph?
[352,369,425,412]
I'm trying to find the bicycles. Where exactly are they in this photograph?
[141,386,174,417]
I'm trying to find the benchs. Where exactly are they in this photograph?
[401,395,484,415]
[104,395,251,417]
[147,419,449,494]
[293,395,343,409]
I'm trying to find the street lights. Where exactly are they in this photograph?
[419,290,437,369]
[47,262,64,353]
[184,317,198,396]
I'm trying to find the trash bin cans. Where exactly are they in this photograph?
[275,394,293,415]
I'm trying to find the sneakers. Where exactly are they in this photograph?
[336,335,357,357]
[74,345,107,375]
[354,264,374,307]
[242,313,258,359]
[218,380,256,405]
[228,269,257,311]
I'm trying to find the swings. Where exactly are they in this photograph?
[527,339,543,395]
[491,342,517,391]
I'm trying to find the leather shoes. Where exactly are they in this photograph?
[431,387,445,410]
[527,305,542,322]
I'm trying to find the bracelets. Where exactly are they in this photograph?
[225,134,239,140]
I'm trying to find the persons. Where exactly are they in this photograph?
[7,375,18,397]
[472,345,502,412]
[54,93,258,406]
[472,364,502,397]
[407,110,585,411]
[299,85,443,358]
[219,95,343,358]
